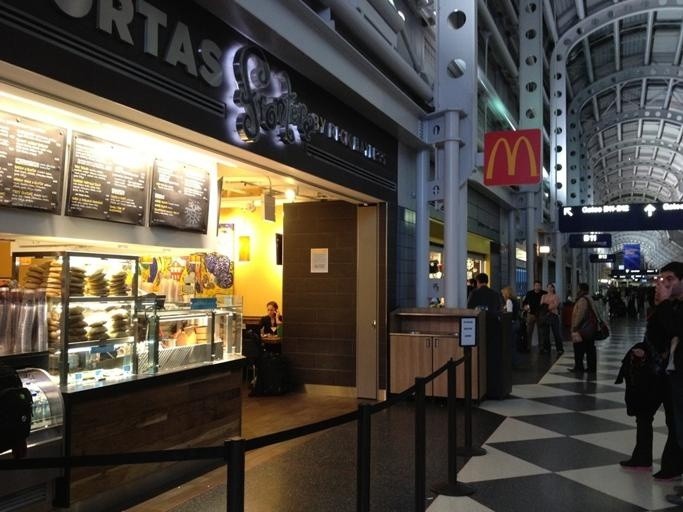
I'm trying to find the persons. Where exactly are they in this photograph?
[257,301,281,351]
[467,271,656,373]
[614,261,681,480]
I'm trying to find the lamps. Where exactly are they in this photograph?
[538,244,550,255]
[283,183,300,203]
[244,199,257,214]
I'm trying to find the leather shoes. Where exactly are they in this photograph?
[566,366,589,373]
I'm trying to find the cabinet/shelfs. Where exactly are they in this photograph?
[1,251,248,511]
[387,305,488,410]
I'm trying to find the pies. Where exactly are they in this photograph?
[47,306,129,345]
[20,259,131,300]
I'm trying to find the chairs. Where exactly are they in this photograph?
[242,328,282,383]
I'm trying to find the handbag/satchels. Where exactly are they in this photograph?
[587,320,612,342]
[577,311,599,339]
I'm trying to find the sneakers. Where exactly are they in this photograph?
[619,457,652,473]
[661,485,683,505]
[651,469,683,483]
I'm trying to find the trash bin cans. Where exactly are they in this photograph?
[562,303,574,327]
